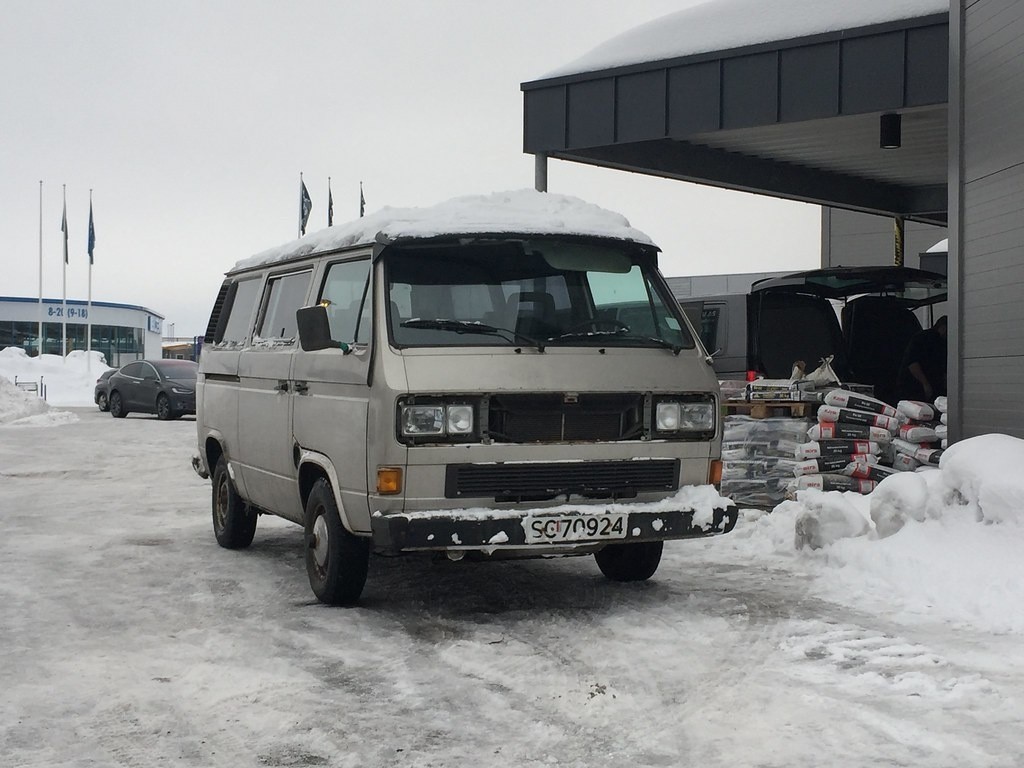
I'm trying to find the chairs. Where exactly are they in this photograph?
[494,290,562,339]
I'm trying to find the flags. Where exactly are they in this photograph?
[328,185,334,227]
[88,202,96,266]
[61,203,69,264]
[360,186,366,218]
[300,179,313,237]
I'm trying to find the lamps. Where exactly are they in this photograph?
[879,114,901,150]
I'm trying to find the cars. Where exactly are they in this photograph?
[94,370,120,413]
[106,358,200,420]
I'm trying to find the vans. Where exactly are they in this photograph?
[476,264,946,407]
[195,197,741,610]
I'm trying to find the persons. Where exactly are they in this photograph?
[894,314,947,409]
